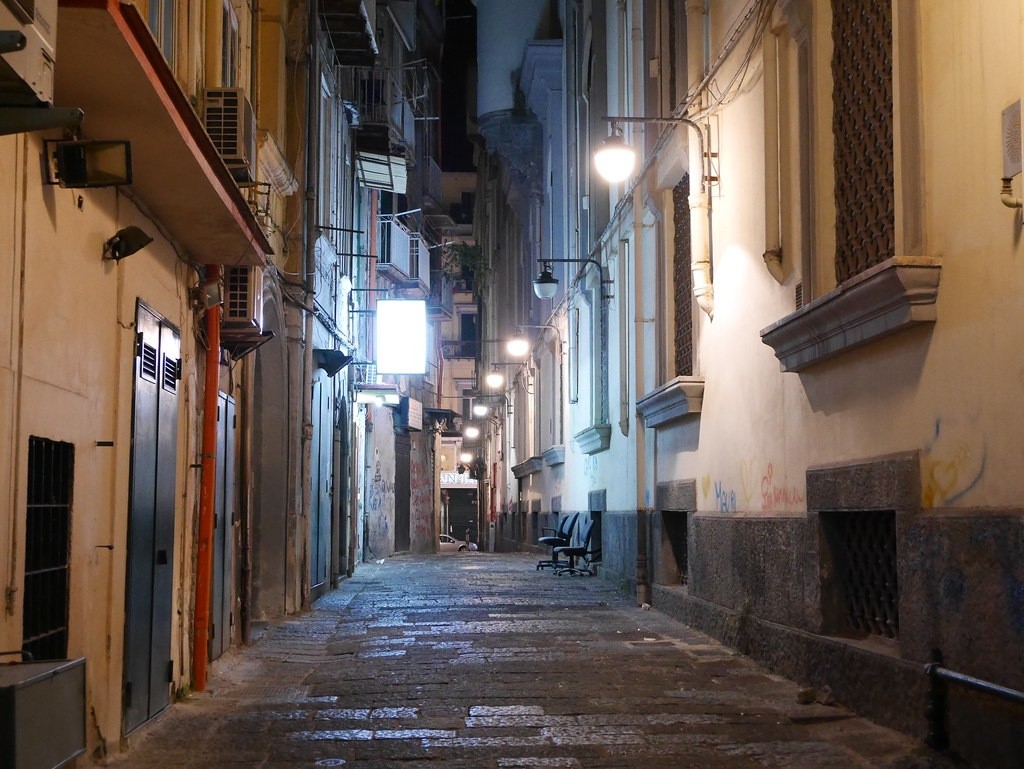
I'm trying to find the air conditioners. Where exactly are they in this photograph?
[203,86,257,183]
[224,264,264,334]
[0,0,59,108]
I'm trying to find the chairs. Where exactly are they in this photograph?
[537,510,594,576]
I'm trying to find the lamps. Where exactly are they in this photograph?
[457,463,475,476]
[189,280,224,310]
[474,113,718,426]
[223,330,275,363]
[103,223,156,263]
[314,349,354,378]
[43,135,134,189]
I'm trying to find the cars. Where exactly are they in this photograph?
[440,534,478,551]
